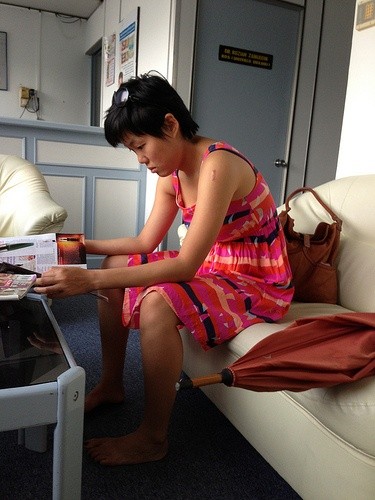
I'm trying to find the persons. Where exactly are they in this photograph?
[34,69,293,466]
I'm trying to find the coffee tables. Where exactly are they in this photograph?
[0,296,86,500]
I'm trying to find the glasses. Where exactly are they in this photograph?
[111,87,138,104]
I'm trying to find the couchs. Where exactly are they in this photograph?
[180,175,375,500]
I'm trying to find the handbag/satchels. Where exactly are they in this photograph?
[280,187,342,304]
